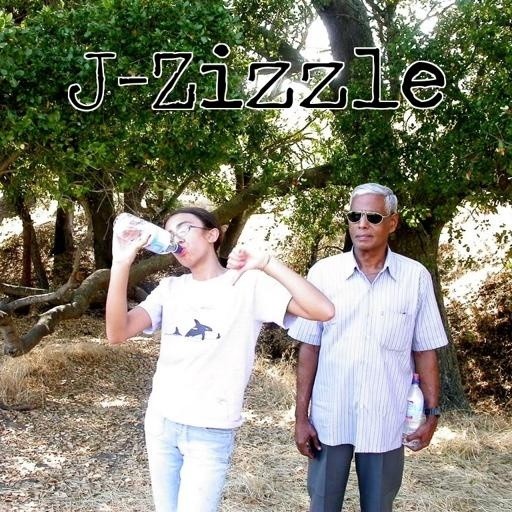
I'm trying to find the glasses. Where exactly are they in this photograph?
[346,211,393,225]
[172,224,211,238]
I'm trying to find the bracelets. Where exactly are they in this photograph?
[259,252,273,272]
[423,404,442,419]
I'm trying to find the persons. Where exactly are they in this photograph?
[104,204,337,512]
[287,181,450,512]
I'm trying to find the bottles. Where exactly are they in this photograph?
[401,373,424,447]
[112,212,183,255]
